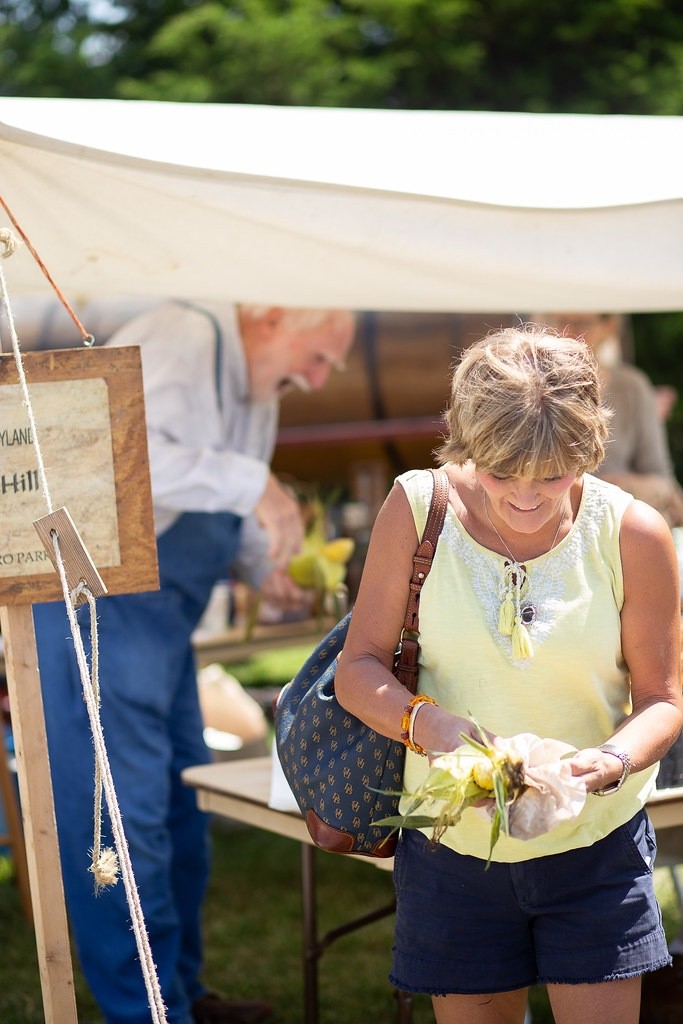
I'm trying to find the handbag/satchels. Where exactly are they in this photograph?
[273,468,447,860]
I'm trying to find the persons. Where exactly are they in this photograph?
[336,327,683,1023]
[535,314,683,526]
[34,302,354,1023]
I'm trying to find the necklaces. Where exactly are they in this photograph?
[482,487,566,625]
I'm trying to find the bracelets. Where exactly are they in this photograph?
[400,696,440,757]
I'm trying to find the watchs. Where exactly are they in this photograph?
[592,745,631,796]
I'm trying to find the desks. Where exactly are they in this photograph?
[180,756,683,1024]
